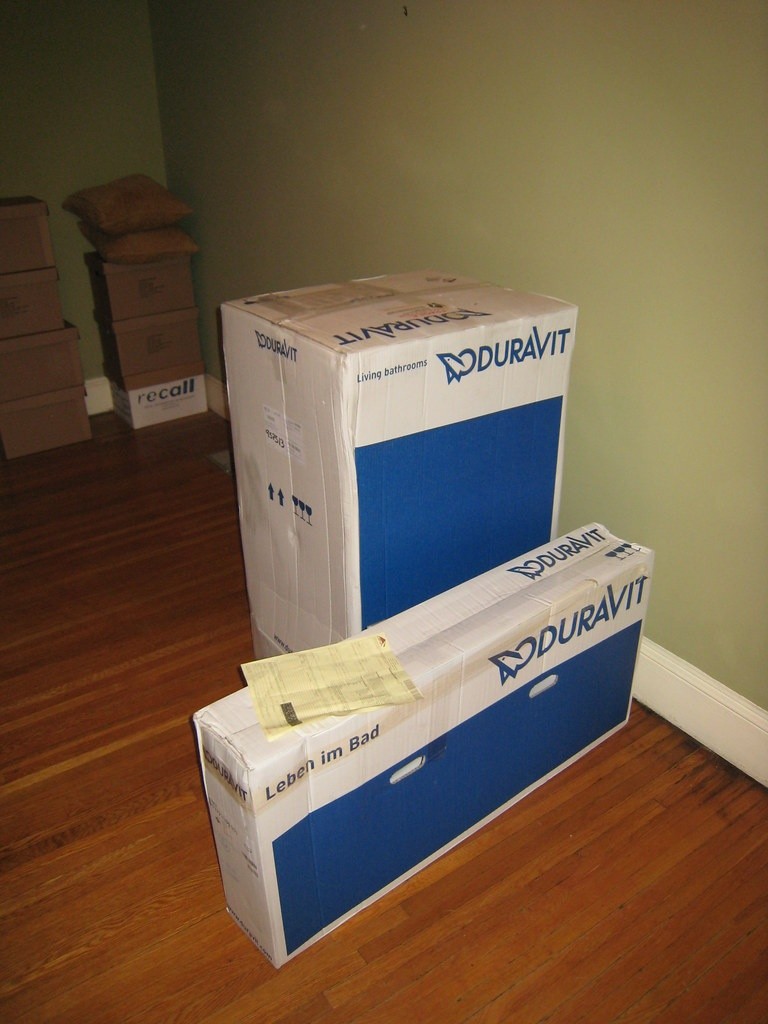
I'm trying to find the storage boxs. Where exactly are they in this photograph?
[192,521,652,972]
[102,257,209,430]
[0,194,92,461]
[219,269,576,660]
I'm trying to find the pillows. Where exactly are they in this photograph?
[63,173,193,236]
[77,221,201,267]
